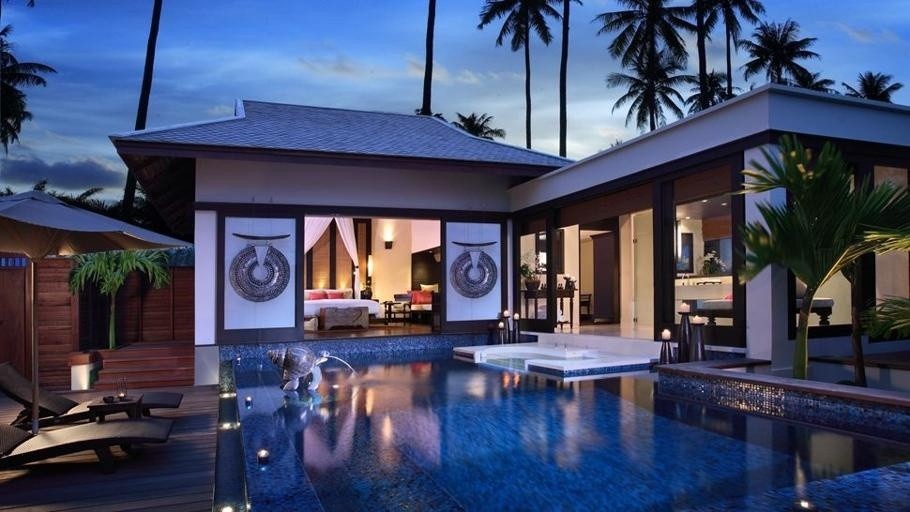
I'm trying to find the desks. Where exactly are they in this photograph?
[521,290,574,329]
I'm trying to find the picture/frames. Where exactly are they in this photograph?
[676,233,694,273]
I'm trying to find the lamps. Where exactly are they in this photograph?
[365,254,372,300]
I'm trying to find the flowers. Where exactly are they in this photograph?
[520,249,543,280]
[702,250,727,274]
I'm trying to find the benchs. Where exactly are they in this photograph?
[697,298,834,326]
[320,307,369,330]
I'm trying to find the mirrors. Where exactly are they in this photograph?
[535,229,565,274]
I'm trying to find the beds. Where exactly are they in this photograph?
[305,288,380,321]
[376,283,439,320]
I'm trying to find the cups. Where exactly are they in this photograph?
[115,376,128,399]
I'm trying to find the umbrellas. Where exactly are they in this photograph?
[0,190,194,434]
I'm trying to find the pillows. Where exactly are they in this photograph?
[406,289,432,304]
[327,292,344,299]
[310,292,327,299]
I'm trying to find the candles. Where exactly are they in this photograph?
[662,303,704,340]
[498,310,519,328]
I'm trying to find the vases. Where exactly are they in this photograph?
[524,280,540,290]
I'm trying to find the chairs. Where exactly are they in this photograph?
[0,363,184,474]
[385,294,413,325]
[580,294,592,319]
